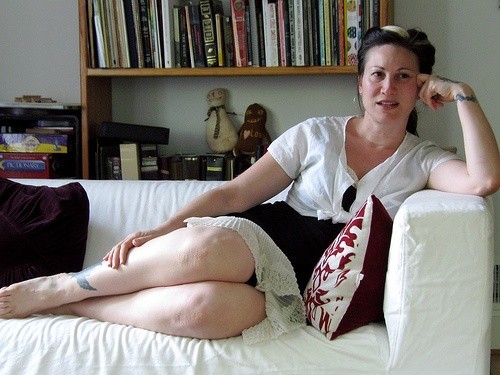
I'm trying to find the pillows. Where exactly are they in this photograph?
[301,194,393,341]
[0,178,90,289]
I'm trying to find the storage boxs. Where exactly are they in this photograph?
[0,134,68,179]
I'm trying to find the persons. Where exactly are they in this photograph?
[0,23,499,341]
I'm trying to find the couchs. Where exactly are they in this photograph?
[0,175,493,375]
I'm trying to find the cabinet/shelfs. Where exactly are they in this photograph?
[78,0,396,180]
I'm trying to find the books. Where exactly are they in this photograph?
[84,0,380,65]
